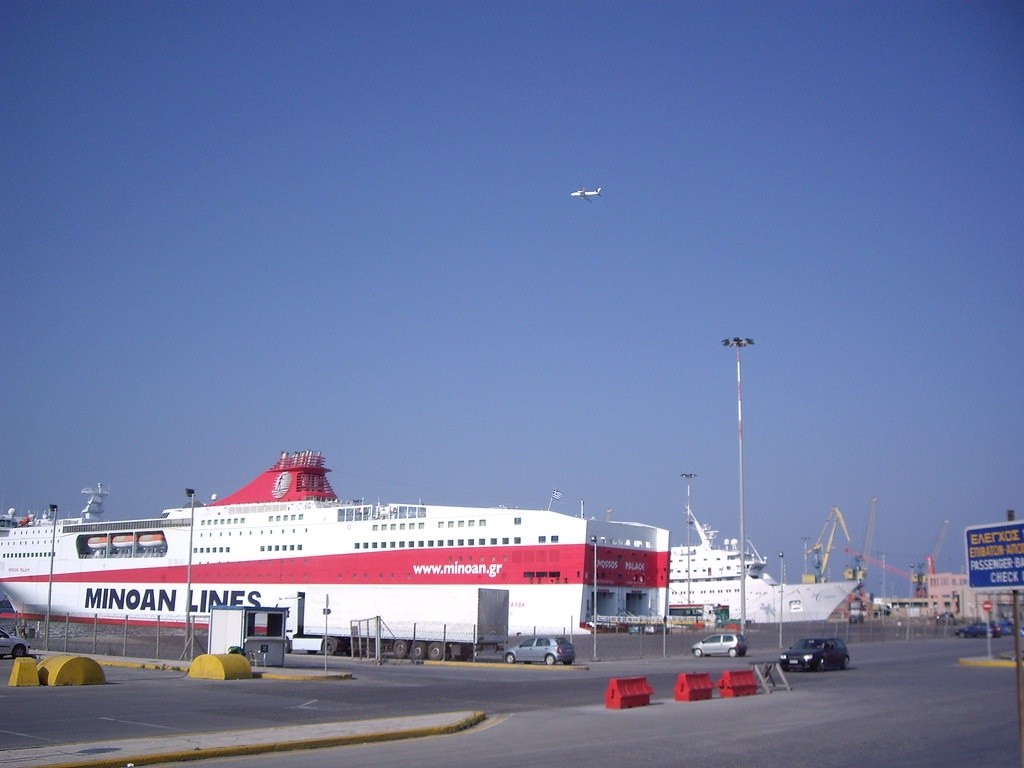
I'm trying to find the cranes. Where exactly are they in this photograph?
[801,508,950,584]
[908,519,953,588]
[801,510,853,582]
[842,496,880,582]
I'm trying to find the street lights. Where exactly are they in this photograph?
[777,547,790,645]
[586,530,602,658]
[681,469,697,608]
[43,503,58,653]
[801,535,810,578]
[183,485,195,659]
[721,333,753,637]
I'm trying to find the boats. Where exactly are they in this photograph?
[668,507,862,624]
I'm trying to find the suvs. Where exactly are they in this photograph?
[781,635,854,673]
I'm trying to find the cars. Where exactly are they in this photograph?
[0,629,31,656]
[992,617,1019,637]
[628,623,644,636]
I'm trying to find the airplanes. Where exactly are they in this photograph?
[569,185,602,203]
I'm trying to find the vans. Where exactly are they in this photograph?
[504,633,575,664]
[951,620,1001,638]
[685,631,746,657]
[646,624,670,636]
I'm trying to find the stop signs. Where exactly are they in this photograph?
[982,600,992,612]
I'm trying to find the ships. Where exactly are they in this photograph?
[0,451,674,659]
